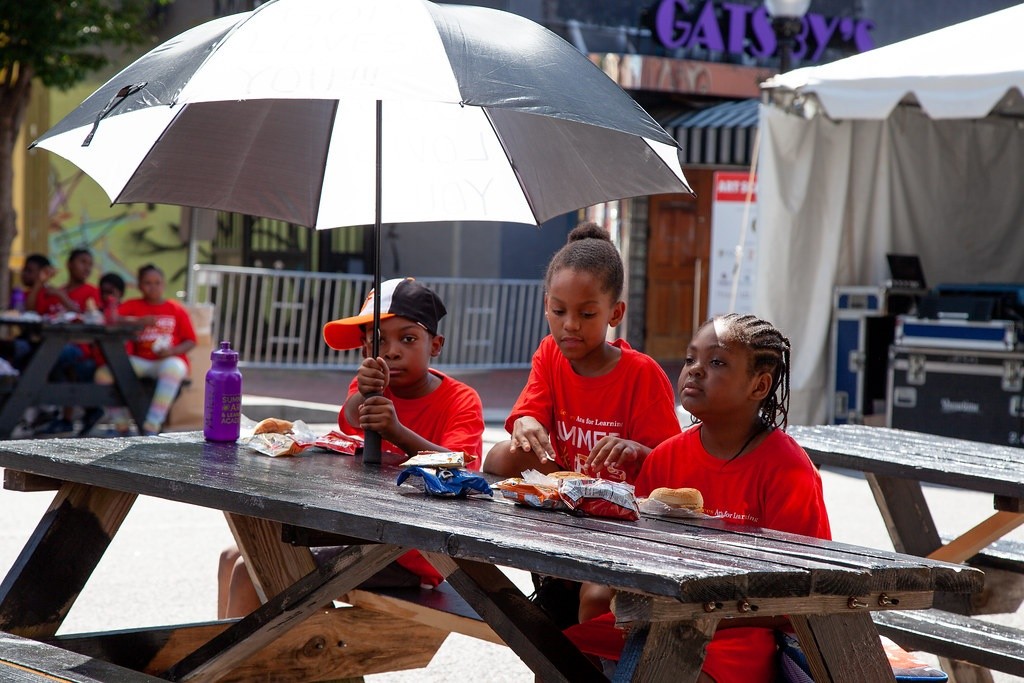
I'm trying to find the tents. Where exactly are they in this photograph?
[756,3,1024,428]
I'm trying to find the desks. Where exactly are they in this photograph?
[0,430,984,683]
[780,424,1024,558]
[1,315,156,443]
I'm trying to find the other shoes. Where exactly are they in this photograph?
[42,419,74,434]
[11,420,35,439]
[78,406,104,437]
[526,573,583,617]
[105,428,131,437]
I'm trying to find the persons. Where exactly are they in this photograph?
[219,276,485,618]
[578,314,833,683]
[482,223,684,627]
[8,249,197,436]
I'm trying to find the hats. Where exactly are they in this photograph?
[323,276,448,351]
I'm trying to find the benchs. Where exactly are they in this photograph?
[935,526,1024,573]
[0,631,178,683]
[865,606,1024,682]
[137,376,192,434]
[317,564,949,683]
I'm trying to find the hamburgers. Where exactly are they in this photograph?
[647,487,704,514]
[253,417,295,436]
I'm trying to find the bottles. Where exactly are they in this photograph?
[203,342,241,442]
[12,285,24,314]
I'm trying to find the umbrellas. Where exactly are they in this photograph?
[28,0,696,467]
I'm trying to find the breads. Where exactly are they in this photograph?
[548,472,588,481]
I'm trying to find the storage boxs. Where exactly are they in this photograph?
[826,286,1024,449]
